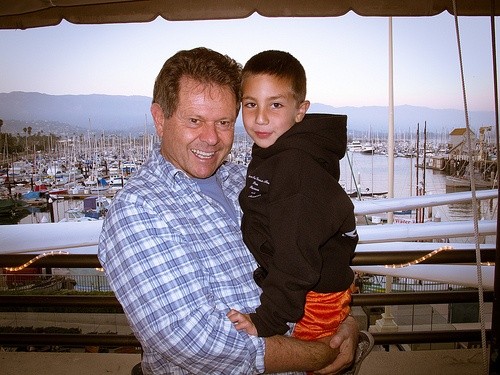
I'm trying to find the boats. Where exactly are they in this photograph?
[0,113,495,295]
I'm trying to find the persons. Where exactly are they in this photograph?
[226,49,358,375]
[98,47,359,375]
